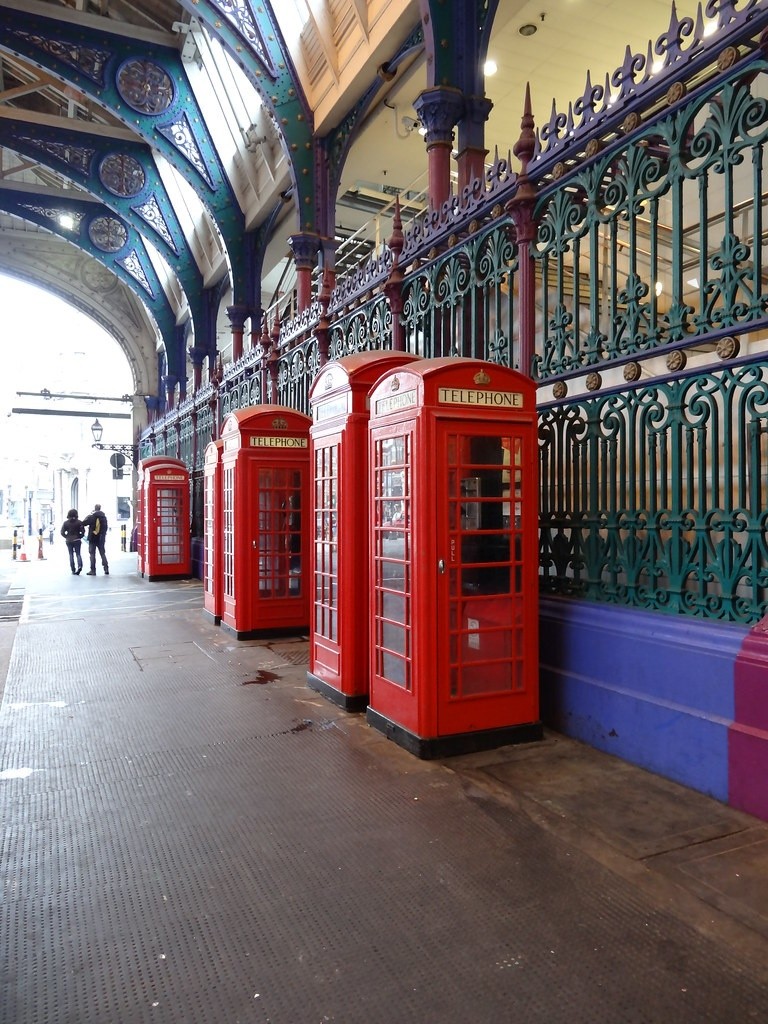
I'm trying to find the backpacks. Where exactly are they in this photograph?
[92,515,107,536]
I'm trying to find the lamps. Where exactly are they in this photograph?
[90,419,138,470]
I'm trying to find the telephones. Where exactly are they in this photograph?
[281,496,294,526]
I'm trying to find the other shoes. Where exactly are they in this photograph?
[72,570,75,575]
[76,569,82,575]
[105,572,109,574]
[86,571,96,576]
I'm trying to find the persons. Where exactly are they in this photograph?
[82,504,109,575]
[61,509,86,575]
[47,522,55,545]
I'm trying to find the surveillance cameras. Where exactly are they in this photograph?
[518,25,537,36]
